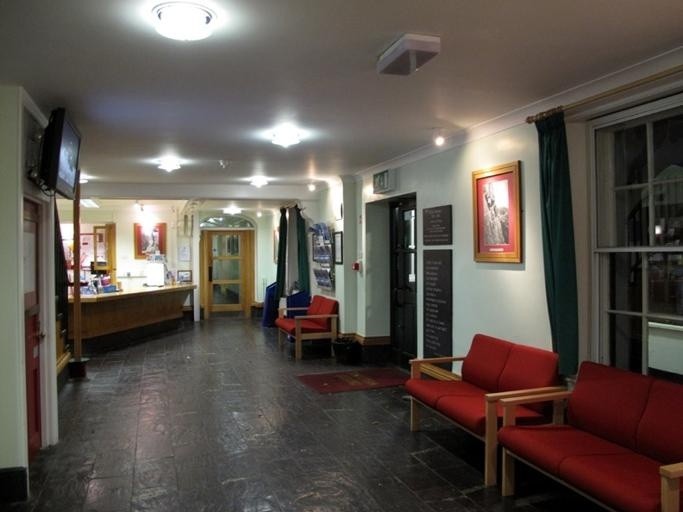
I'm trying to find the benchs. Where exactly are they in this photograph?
[499,359,682,511]
[402,332,569,484]
[274,294,338,362]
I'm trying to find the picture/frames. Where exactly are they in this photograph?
[132,222,167,260]
[176,269,192,282]
[470,160,521,264]
[332,231,343,264]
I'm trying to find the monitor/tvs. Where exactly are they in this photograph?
[40,108,81,199]
[90,262,106,278]
[177,270,192,283]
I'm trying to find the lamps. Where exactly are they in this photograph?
[150,2,217,43]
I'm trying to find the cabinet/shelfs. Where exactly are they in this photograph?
[93,223,115,284]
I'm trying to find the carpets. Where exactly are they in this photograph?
[292,364,411,394]
